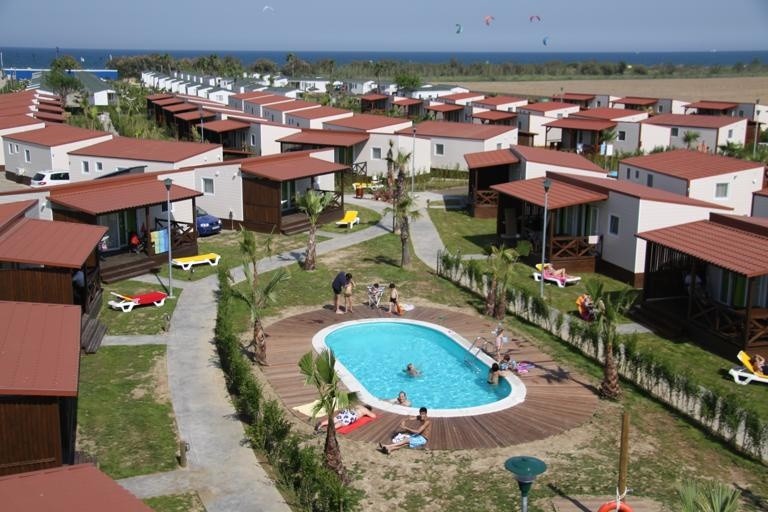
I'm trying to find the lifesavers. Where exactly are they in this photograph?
[598,500,634,512]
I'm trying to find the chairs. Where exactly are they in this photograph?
[532,262,581,289]
[106,291,169,312]
[171,252,221,271]
[352,183,371,191]
[336,211,360,229]
[728,350,768,386]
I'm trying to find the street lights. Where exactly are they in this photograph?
[752,109,762,160]
[503,455,547,512]
[539,179,552,301]
[412,127,416,193]
[164,177,176,300]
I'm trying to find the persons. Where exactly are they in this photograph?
[332,271,352,315]
[498,355,511,371]
[314,404,376,431]
[378,406,433,456]
[683,267,706,300]
[385,283,400,314]
[583,295,607,315]
[749,352,768,374]
[486,362,508,385]
[545,264,568,278]
[393,391,410,407]
[526,229,541,253]
[407,363,418,377]
[340,278,355,314]
[71,269,86,304]
[496,327,506,357]
[368,283,381,306]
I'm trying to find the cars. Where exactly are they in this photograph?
[196,204,222,236]
